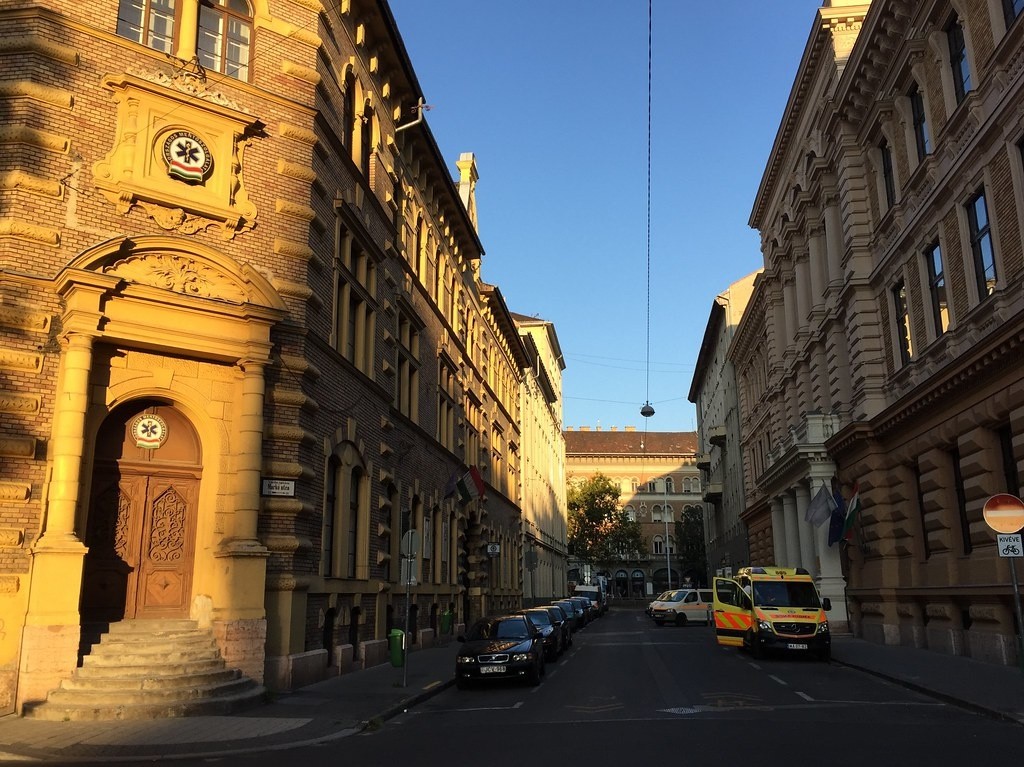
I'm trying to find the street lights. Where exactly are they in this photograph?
[653,475,676,590]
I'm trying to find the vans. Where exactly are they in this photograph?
[645,587,732,627]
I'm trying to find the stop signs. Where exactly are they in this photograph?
[983,492,1024,535]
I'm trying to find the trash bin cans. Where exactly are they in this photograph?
[388,628,405,668]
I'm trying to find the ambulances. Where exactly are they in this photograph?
[711,562,833,661]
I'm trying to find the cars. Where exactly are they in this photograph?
[454,614,547,692]
[510,585,609,662]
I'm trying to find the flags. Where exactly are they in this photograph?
[804,484,838,528]
[841,482,867,554]
[828,476,848,548]
